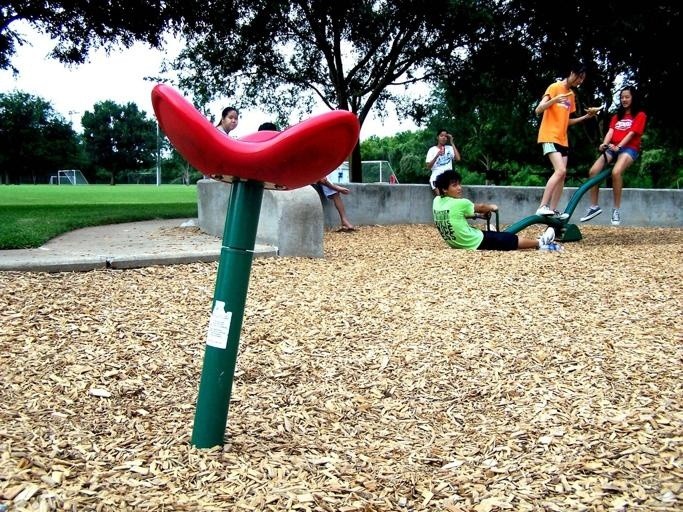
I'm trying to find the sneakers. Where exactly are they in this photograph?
[547,209,570,220]
[535,206,555,217]
[580,206,603,222]
[537,226,555,249]
[610,208,622,226]
[485,223,497,232]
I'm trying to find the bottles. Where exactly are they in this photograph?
[540,243,565,252]
[337,167,343,183]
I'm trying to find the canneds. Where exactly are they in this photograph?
[389,174,395,185]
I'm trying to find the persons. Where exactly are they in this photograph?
[257,122,277,130]
[432,170,555,249]
[215,107,238,135]
[580,86,648,226]
[318,177,354,230]
[534,64,596,220]
[426,129,461,196]
